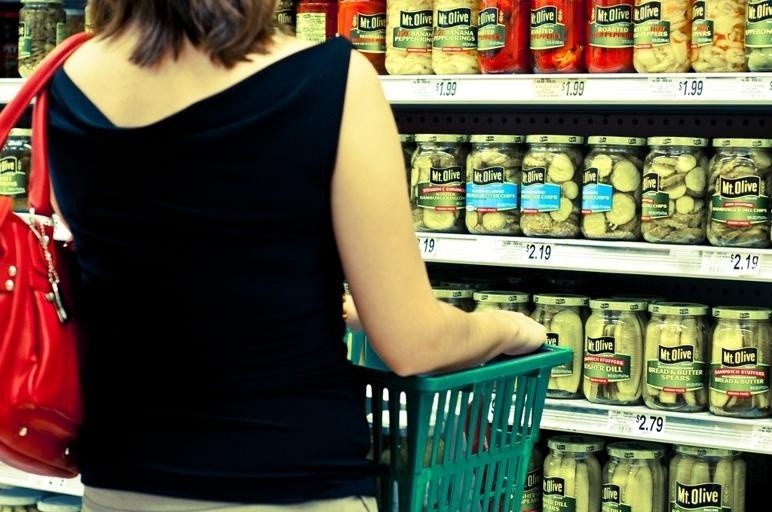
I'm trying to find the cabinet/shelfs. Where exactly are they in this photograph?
[0,1,772,512]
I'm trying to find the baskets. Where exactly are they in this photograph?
[327,319,579,512]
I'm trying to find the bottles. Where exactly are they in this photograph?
[17,1,69,78]
[1,488,42,512]
[607,441,668,511]
[440,279,771,420]
[364,406,450,470]
[542,434,602,511]
[670,444,744,511]
[400,135,770,248]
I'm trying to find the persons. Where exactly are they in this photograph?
[36,0,548,512]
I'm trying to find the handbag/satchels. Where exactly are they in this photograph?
[0,195,81,483]
